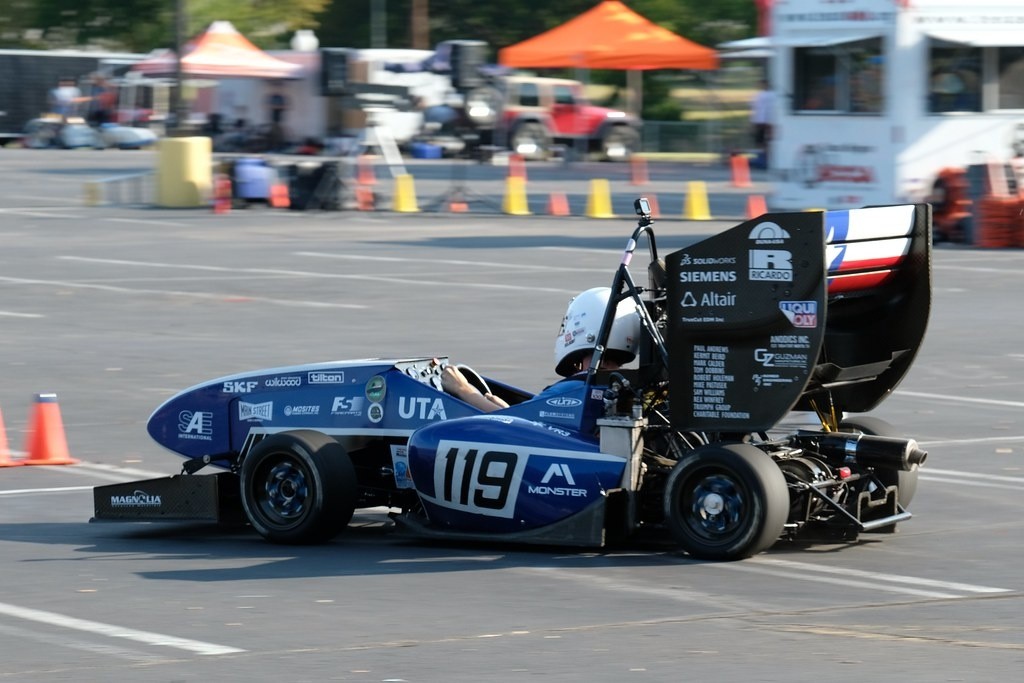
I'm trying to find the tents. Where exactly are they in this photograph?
[131,20,309,83]
[498,0,717,162]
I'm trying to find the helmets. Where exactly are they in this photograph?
[554,287,640,377]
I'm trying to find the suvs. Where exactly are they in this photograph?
[465,75,645,164]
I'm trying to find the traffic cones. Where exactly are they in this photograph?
[394,174,420,213]
[503,155,768,221]
[0,393,80,467]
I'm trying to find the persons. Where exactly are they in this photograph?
[442,286,640,414]
[752,81,769,151]
